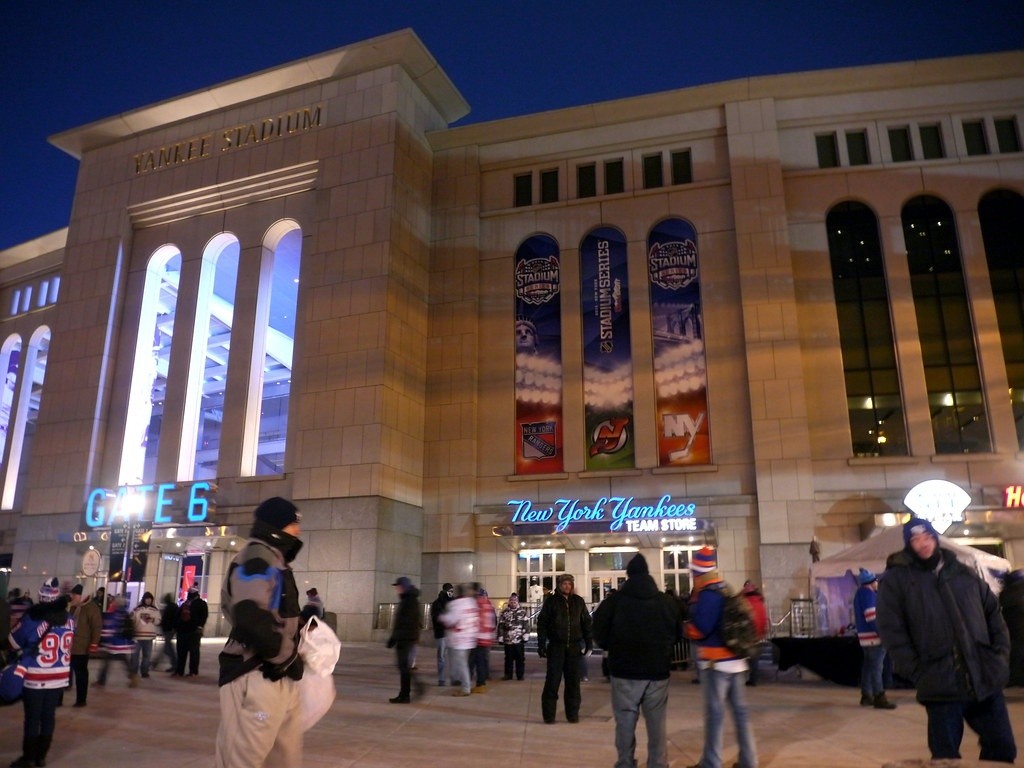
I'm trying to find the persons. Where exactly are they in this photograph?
[853,568,1024,710]
[682,548,759,768]
[591,556,683,768]
[215,496,327,768]
[306,574,765,725]
[875,518,1017,763]
[0,575,208,768]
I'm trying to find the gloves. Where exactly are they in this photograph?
[583,642,594,655]
[520,639,528,643]
[285,656,305,680]
[196,627,204,636]
[88,644,98,655]
[498,641,504,645]
[537,646,547,658]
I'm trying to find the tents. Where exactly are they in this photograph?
[814,524,1013,637]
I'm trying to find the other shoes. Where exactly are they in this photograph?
[128,673,137,687]
[438,679,485,696]
[389,697,410,703]
[90,681,106,688]
[860,695,897,710]
[10,757,46,768]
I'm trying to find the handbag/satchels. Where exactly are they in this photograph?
[0,662,27,706]
[299,617,341,729]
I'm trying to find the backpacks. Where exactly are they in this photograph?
[699,580,757,658]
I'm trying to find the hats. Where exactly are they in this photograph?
[902,518,938,542]
[189,581,198,593]
[689,546,718,573]
[71,583,84,594]
[443,583,453,591]
[306,587,317,595]
[558,574,574,596]
[858,568,876,584]
[254,497,300,531]
[627,554,649,575]
[39,577,61,597]
[509,593,519,602]
[142,591,153,600]
[392,576,411,586]
